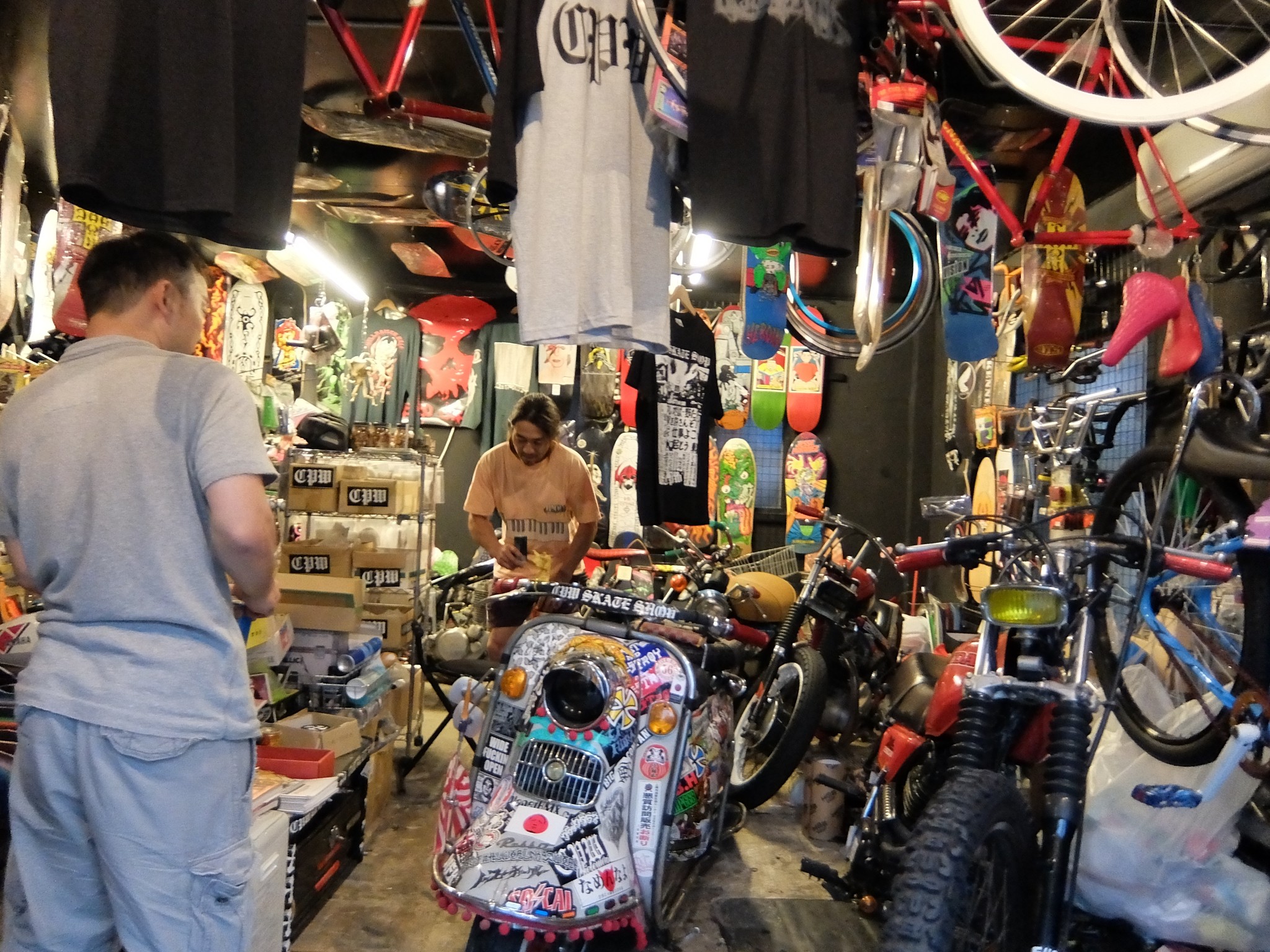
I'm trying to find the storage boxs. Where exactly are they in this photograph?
[231,463,444,952]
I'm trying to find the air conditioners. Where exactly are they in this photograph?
[1136,89,1270,222]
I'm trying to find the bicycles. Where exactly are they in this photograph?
[786,1,1270,758]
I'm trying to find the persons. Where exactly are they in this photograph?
[466,392,600,666]
[0,235,286,952]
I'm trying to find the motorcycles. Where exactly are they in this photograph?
[403,497,1233,952]
